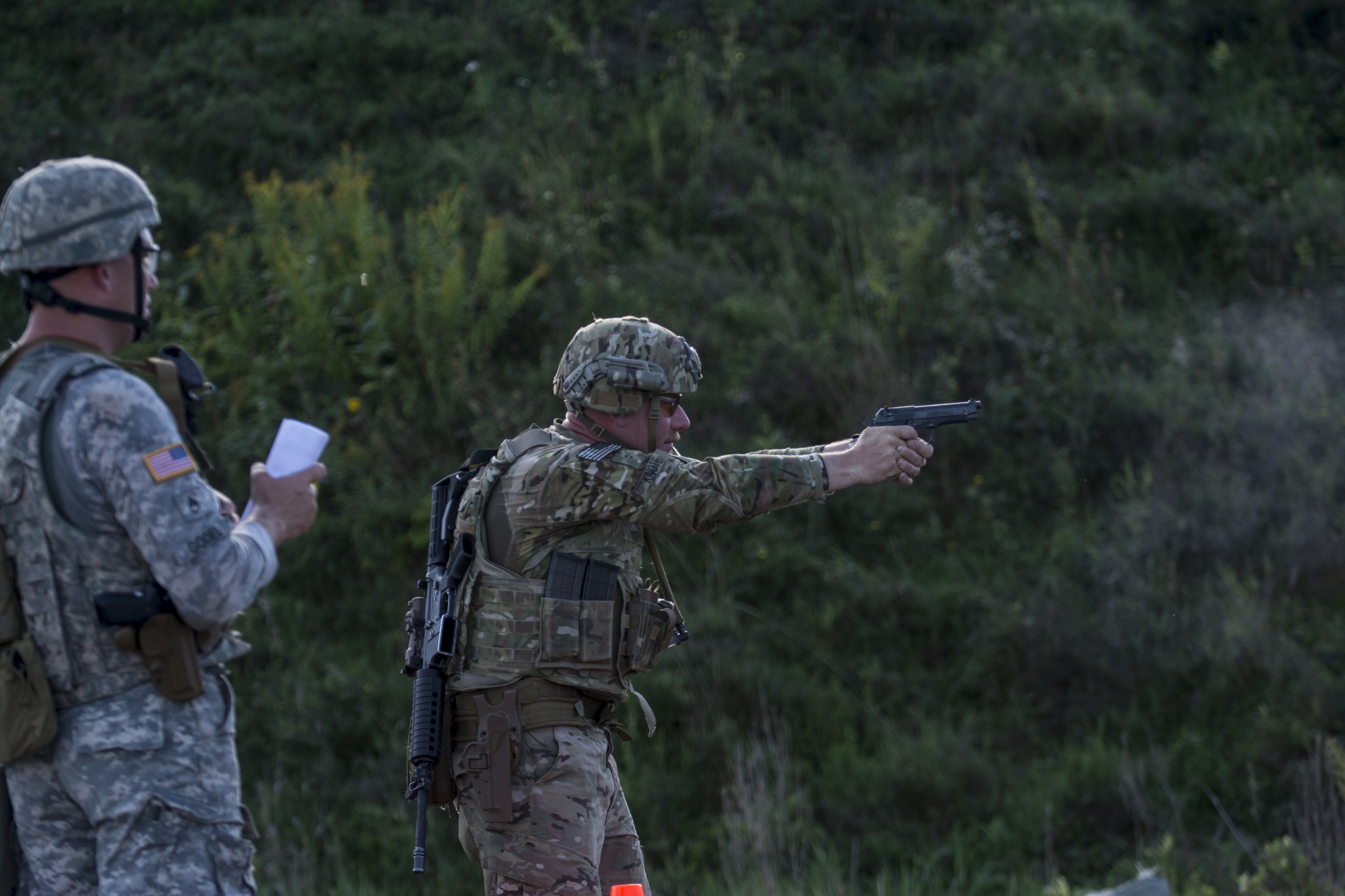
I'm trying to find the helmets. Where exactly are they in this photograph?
[552,316,702,416]
[0,154,162,274]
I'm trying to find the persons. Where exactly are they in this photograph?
[442,318,933,896]
[1,154,326,896]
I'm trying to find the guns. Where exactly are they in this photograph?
[869,400,985,458]
[395,472,472,878]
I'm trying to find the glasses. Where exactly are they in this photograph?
[649,393,683,417]
[128,243,160,274]
